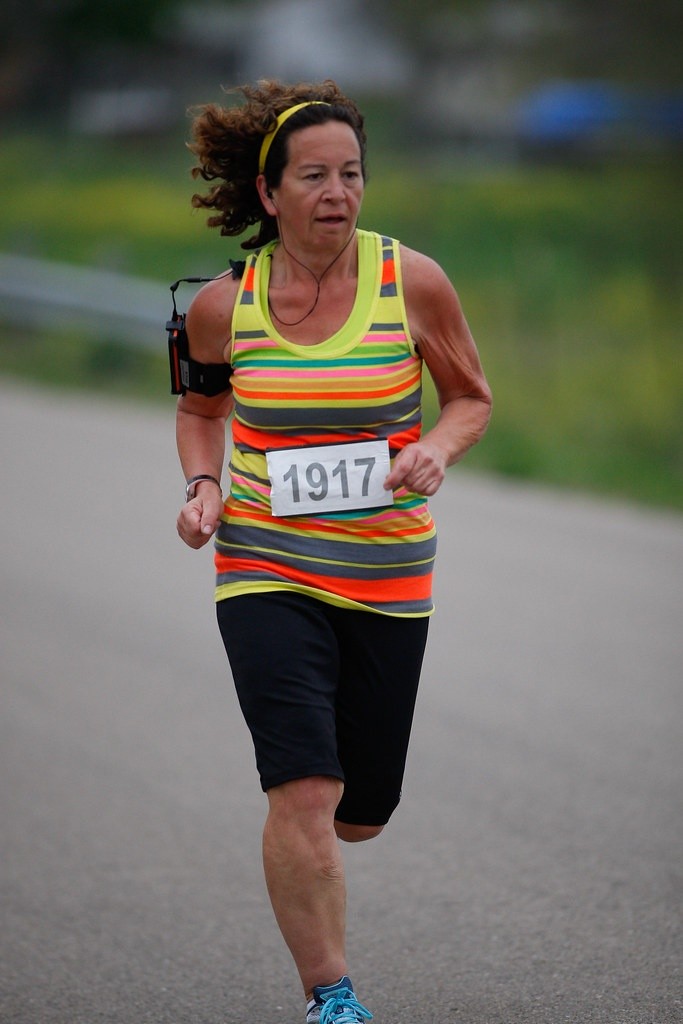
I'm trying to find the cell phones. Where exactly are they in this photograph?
[168,315,184,394]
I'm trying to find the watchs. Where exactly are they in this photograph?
[184,475,224,505]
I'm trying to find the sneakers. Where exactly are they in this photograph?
[304,977,366,1024]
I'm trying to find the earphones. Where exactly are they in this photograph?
[266,190,272,199]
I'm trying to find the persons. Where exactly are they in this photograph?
[167,84,490,1024]
